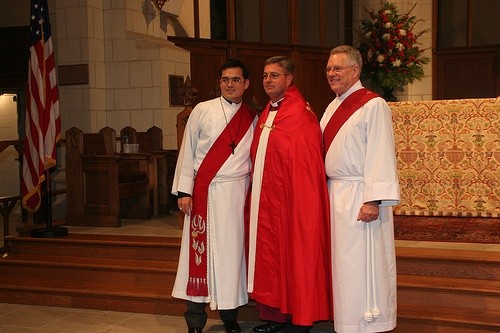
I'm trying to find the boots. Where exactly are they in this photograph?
[184,311,207,333]
[219,308,241,333]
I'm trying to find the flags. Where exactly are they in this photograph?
[22,0,63,212]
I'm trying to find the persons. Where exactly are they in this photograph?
[172,60,262,333]
[243,56,333,333]
[319,46,401,333]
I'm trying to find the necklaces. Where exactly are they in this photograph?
[219,95,243,155]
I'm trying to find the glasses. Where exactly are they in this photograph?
[263,73,289,79]
[219,78,245,84]
[324,66,353,74]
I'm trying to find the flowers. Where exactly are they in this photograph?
[353,0,432,98]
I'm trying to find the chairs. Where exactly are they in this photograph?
[64,124,155,229]
[119,126,182,217]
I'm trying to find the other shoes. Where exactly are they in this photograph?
[253,321,313,333]
